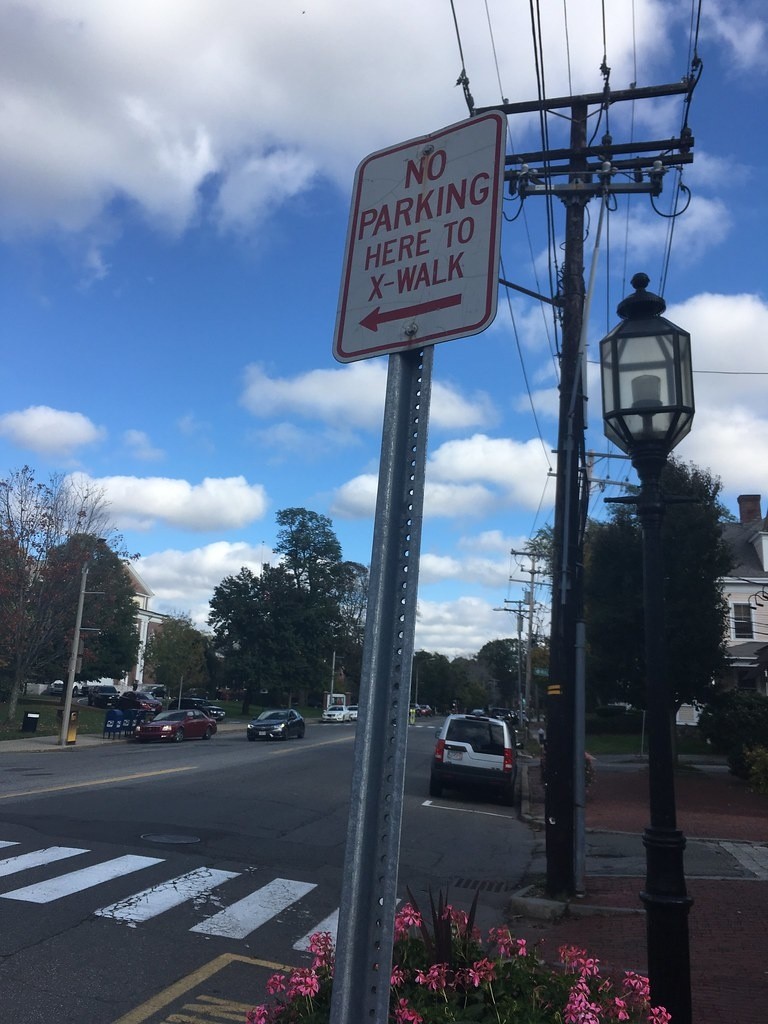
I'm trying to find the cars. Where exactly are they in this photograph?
[140,684,165,700]
[49,680,85,697]
[430,712,525,806]
[116,691,163,715]
[88,685,121,707]
[247,708,306,741]
[322,700,531,727]
[135,708,218,743]
[167,698,227,722]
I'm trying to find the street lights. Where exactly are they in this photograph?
[597,269,700,1024]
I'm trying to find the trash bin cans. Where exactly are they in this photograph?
[102,708,160,741]
[55,707,80,745]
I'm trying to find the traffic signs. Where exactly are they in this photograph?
[332,109,508,364]
